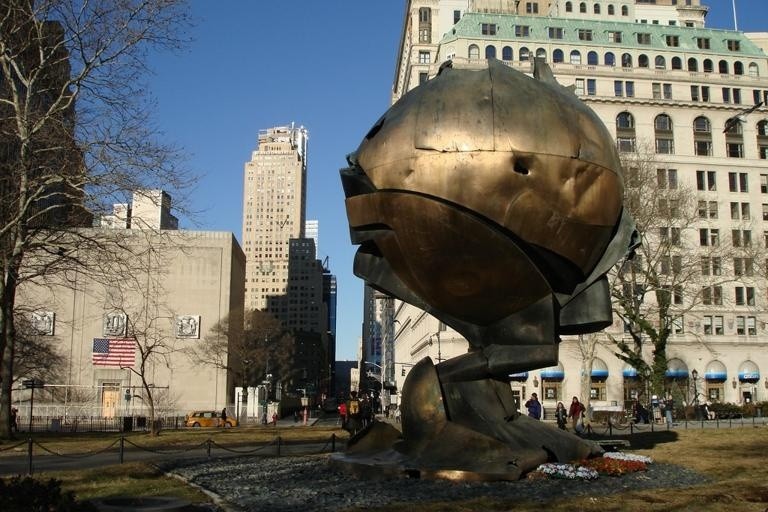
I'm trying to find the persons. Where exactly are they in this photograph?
[260,385,408,435]
[663,393,674,431]
[221,407,229,428]
[525,392,542,421]
[554,401,568,431]
[568,395,587,433]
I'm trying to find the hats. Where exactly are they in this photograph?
[532,393,537,398]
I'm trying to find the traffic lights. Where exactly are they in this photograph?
[401,369,405,376]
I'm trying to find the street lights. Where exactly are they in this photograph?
[364,361,383,414]
[295,388,306,425]
[690,368,699,421]
[22,376,45,477]
[643,364,652,407]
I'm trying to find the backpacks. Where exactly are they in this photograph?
[348,398,360,418]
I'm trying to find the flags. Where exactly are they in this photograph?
[93,338,138,367]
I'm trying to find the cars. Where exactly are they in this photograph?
[183,410,238,430]
